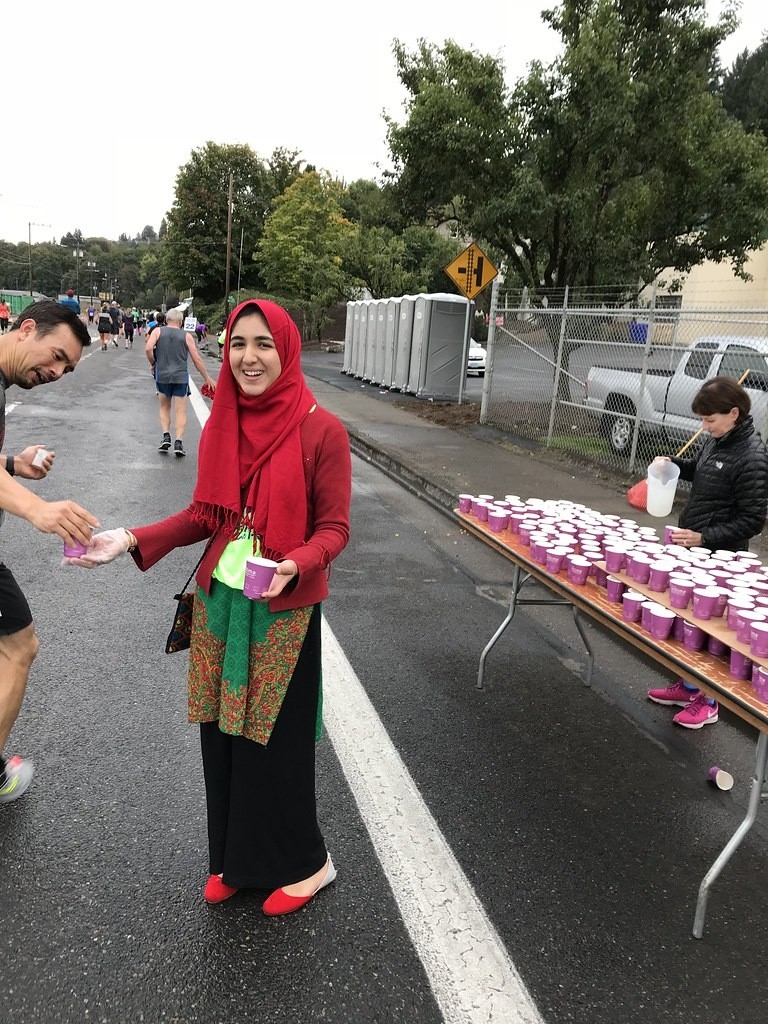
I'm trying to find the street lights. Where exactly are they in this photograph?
[87,261,95,305]
[73,250,83,301]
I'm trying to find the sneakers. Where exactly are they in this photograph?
[175,442,186,456]
[158,435,171,450]
[648,679,701,708]
[0,756,34,803]
[673,689,718,729]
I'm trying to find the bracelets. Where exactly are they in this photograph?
[6,454,15,477]
[125,530,136,553]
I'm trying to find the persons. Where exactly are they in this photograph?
[65,300,351,917]
[87,301,168,351]
[647,375,768,729]
[0,301,92,803]
[146,308,217,454]
[218,329,226,357]
[195,324,211,344]
[0,299,11,335]
[60,290,81,315]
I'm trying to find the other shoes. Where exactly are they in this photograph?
[102,343,107,350]
[262,850,337,917]
[204,873,240,904]
[112,340,119,347]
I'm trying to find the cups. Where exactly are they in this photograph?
[64,526,93,558]
[243,557,279,599]
[31,449,50,470]
[709,766,734,790]
[458,494,768,704]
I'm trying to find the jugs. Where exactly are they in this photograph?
[646,459,681,517]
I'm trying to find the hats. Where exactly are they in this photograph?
[67,289,74,295]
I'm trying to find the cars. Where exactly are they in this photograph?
[467,337,486,378]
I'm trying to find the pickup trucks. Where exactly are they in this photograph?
[582,337,768,457]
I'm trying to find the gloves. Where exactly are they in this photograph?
[165,591,195,654]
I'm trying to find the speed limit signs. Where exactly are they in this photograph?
[183,317,197,332]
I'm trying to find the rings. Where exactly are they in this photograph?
[684,539,687,544]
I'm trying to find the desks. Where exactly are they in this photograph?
[453,508,768,940]
